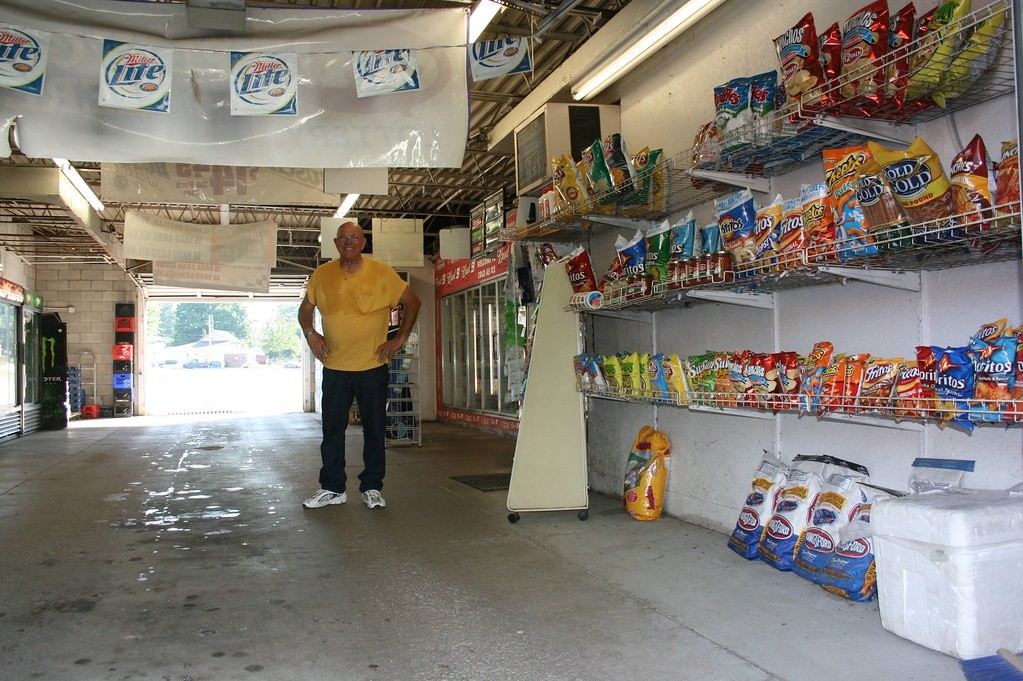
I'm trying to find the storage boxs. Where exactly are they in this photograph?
[110,344,134,417]
[869,490,1023,662]
[68,364,85,412]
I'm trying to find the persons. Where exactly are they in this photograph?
[298,220,422,509]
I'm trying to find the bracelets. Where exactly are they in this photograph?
[303,327,314,338]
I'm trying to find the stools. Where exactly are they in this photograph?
[83,404,100,418]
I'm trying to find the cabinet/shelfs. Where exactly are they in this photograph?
[506,5,1022,435]
[382,353,420,446]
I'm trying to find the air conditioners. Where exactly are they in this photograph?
[514,103,620,196]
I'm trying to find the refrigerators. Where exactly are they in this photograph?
[0,276,25,443]
[22,288,44,436]
[433,203,524,440]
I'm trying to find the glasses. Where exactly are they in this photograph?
[336,235,365,243]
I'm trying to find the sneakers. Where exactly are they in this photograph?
[361,489,386,510]
[303,489,347,509]
